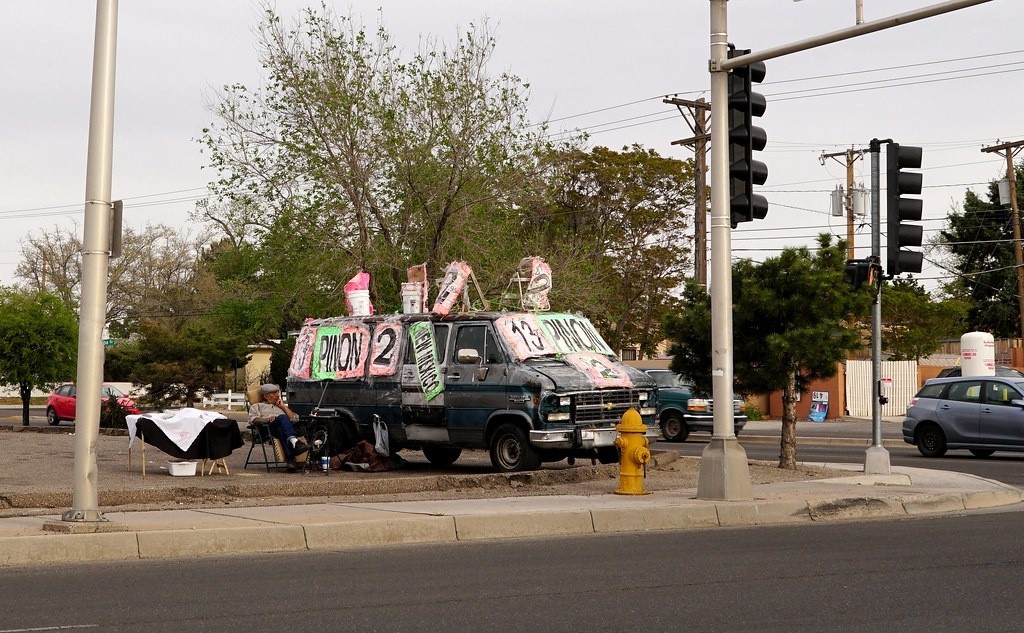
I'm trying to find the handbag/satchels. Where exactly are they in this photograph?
[372,414,389,457]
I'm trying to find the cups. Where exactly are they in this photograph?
[321,456,330,472]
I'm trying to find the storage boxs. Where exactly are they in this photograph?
[167,459,198,477]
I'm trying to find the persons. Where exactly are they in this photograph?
[247,384,311,472]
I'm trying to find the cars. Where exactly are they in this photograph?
[902,366,1024,458]
[46,383,140,427]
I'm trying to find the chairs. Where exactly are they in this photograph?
[244,384,316,473]
[990,390,1001,400]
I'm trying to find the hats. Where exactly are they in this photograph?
[260,384,279,394]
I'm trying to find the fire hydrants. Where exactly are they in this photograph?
[614,404,652,495]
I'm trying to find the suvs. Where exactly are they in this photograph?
[637,367,748,441]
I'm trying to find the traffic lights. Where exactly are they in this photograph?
[887,142,924,278]
[730,49,768,227]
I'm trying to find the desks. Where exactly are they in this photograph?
[124,414,246,476]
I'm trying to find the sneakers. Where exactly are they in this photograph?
[293,439,311,456]
[287,462,297,471]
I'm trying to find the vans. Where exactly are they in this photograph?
[284,311,659,472]
